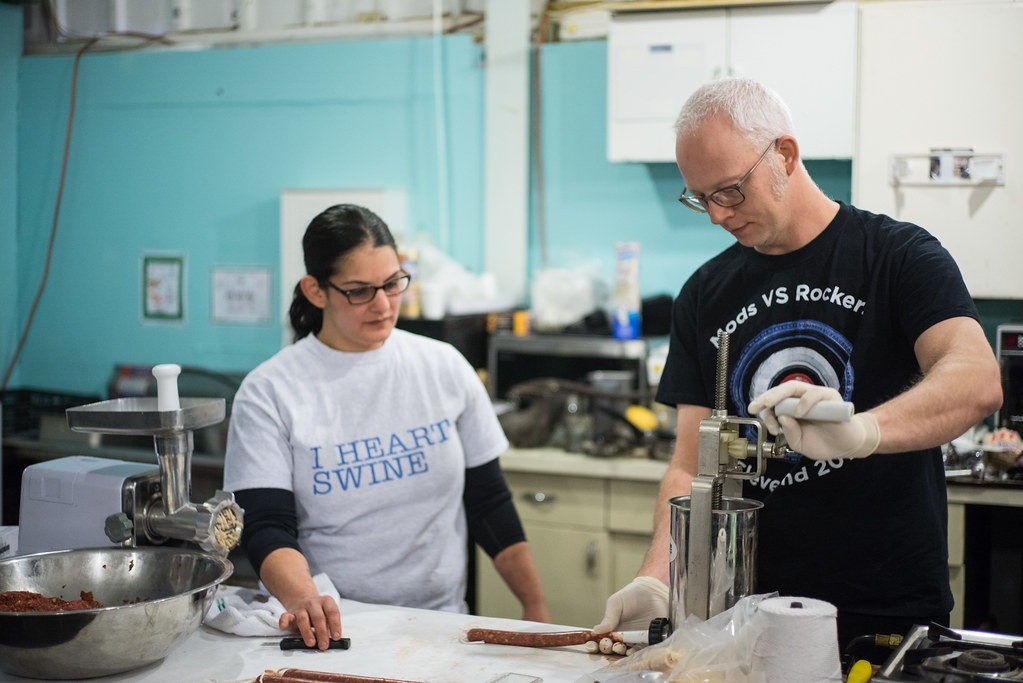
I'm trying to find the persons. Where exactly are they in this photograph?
[219,204,551,651]
[583,78,1003,663]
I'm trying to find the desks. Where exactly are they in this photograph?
[0,581,916,683]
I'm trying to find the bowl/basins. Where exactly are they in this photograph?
[1,548,235,677]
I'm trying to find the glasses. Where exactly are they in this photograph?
[319,266,411,305]
[679,137,778,212]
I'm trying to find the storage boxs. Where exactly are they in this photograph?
[0,545,235,682]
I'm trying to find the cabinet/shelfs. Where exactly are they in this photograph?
[848,0,1023,300]
[475,448,1023,633]
[606,0,858,165]
